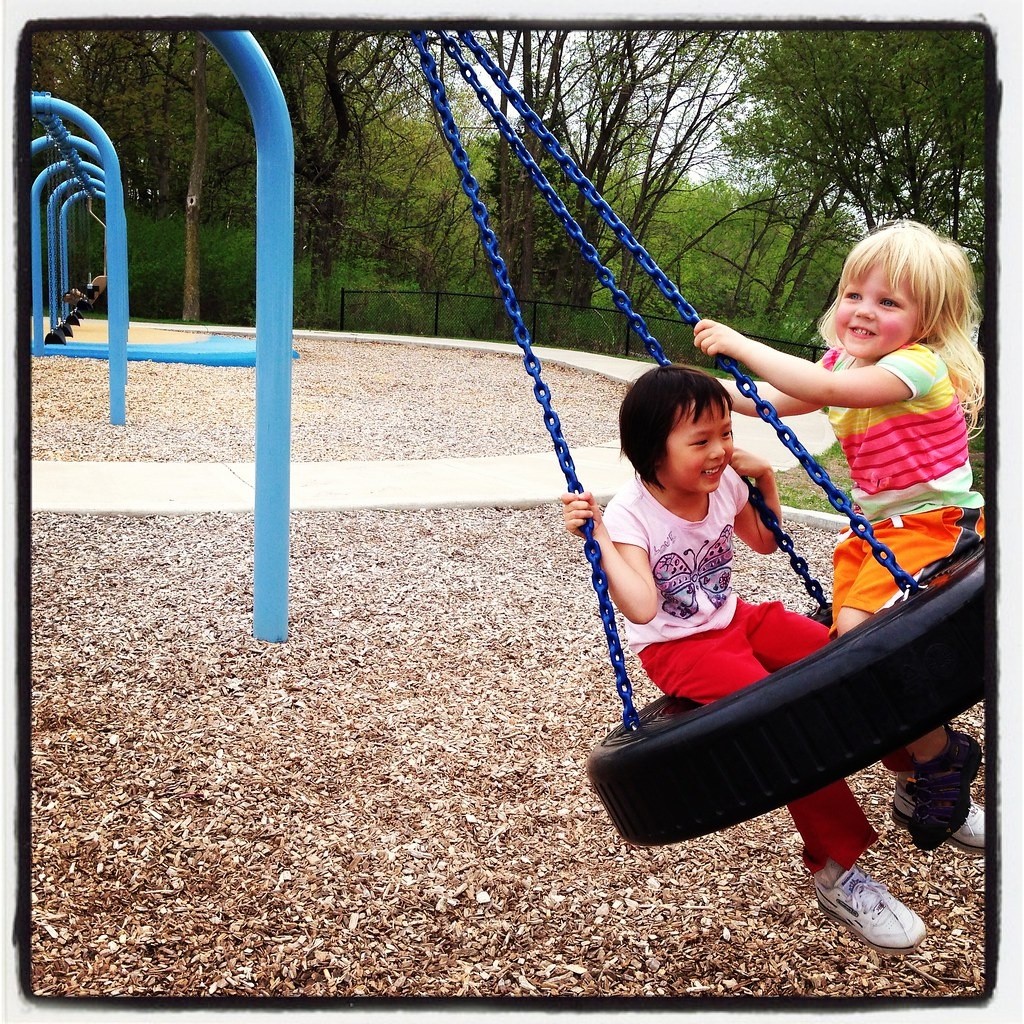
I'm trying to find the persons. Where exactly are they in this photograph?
[562,361,984,954]
[689,216,984,853]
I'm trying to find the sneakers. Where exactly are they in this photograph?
[893,770,985,853]
[910,727,981,850]
[816,864,926,954]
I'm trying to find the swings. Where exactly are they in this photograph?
[413,30,984,846]
[44,126,96,347]
